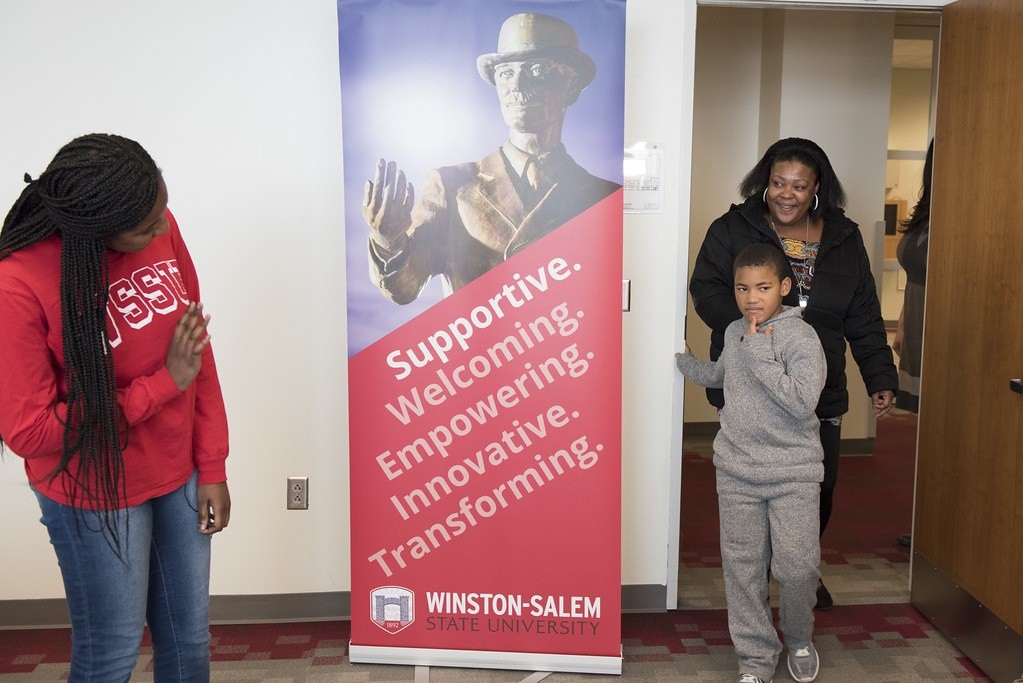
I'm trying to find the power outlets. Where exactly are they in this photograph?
[287,477,308,510]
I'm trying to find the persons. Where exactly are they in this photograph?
[0,132,232,683]
[688,138,898,611]
[892,136,934,547]
[675,244,827,683]
[362,12,624,305]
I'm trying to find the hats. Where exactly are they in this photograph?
[476,13,596,92]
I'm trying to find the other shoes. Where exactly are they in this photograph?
[896,535,911,545]
[813,578,833,610]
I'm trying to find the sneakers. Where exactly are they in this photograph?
[788,641,819,683]
[736,674,772,683]
